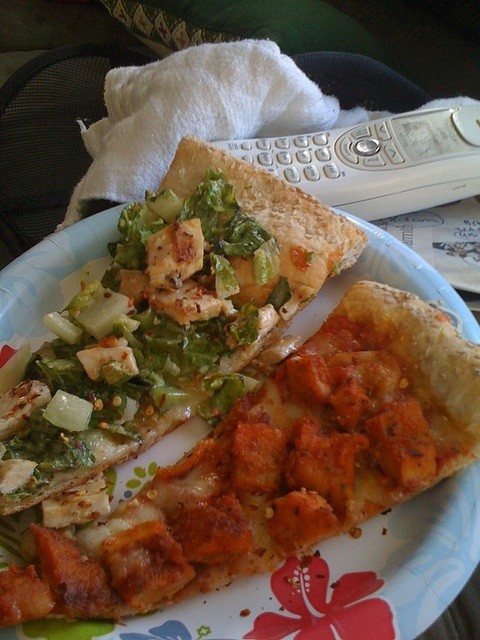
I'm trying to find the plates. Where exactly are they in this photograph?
[0,198,480,640]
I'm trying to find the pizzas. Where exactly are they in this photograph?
[1,279,480,629]
[1,135,367,521]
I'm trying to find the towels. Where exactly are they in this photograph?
[55,39,479,231]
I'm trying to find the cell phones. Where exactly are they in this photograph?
[212,105,479,222]
